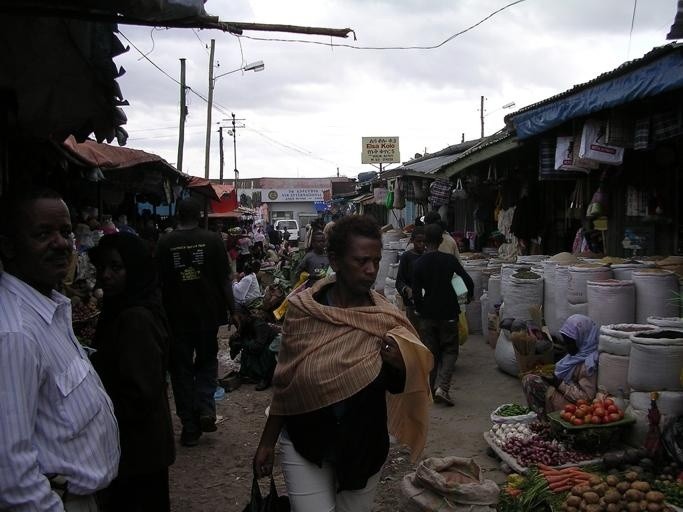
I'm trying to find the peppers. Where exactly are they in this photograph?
[495,404,530,416]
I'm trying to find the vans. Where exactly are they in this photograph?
[273,219,300,247]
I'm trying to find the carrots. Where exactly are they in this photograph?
[539,464,591,492]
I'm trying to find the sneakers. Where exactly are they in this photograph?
[241,376,259,384]
[256,377,270,391]
[181,415,217,447]
[435,387,455,406]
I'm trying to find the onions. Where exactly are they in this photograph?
[502,436,590,465]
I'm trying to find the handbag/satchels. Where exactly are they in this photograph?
[243,473,290,512]
[430,180,451,210]
[451,179,469,202]
[538,108,682,181]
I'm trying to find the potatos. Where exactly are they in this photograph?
[562,472,675,512]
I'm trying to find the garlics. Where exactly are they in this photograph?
[489,423,531,445]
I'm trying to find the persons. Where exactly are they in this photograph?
[227,210,348,333]
[396,210,475,410]
[226,300,282,391]
[89,230,176,512]
[249,212,434,511]
[519,313,600,418]
[1,183,123,512]
[134,208,174,243]
[151,197,236,448]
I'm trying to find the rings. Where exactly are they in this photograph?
[383,344,389,350]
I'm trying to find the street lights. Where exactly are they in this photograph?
[205,60,264,179]
[481,101,515,138]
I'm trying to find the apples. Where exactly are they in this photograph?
[560,399,624,426]
